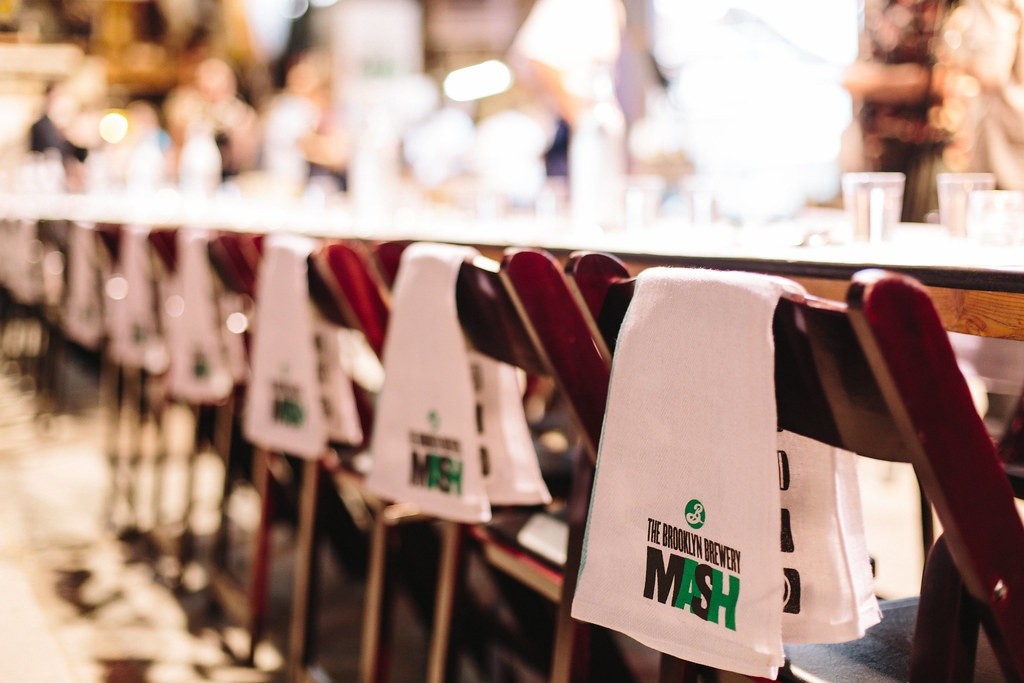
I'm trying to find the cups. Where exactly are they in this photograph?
[969,190,1024,266]
[842,172,906,244]
[936,173,996,237]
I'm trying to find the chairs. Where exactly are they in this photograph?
[1,219,1023,682]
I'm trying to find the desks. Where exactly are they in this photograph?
[1,197,1022,287]
